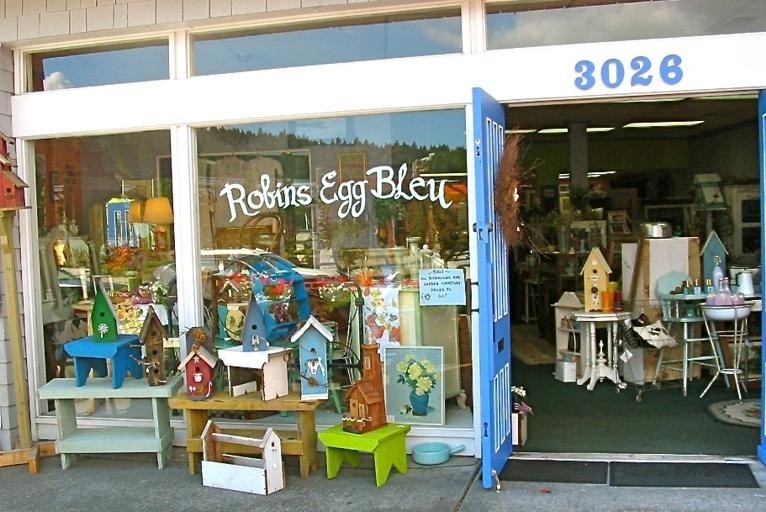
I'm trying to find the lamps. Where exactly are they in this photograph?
[141,197,173,251]
[128,201,150,223]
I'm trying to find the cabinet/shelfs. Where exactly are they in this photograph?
[620,235,702,384]
[168,385,324,479]
[342,280,468,414]
[514,177,764,320]
[37,374,181,470]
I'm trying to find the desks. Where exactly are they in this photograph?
[572,310,631,391]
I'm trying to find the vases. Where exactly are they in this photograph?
[409,391,429,416]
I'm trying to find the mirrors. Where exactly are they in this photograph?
[51,236,94,301]
[104,197,151,251]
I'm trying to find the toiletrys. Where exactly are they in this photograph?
[684,275,733,296]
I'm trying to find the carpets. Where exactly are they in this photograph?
[608,457,761,488]
[500,456,609,486]
[706,398,762,427]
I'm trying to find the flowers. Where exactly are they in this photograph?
[395,353,440,396]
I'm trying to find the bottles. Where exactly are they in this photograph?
[602,279,623,311]
[713,256,730,294]
[682,279,714,294]
[408,242,444,284]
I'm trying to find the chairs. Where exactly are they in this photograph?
[653,272,730,397]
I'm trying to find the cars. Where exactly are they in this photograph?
[55,270,129,302]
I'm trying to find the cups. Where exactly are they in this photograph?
[379,265,399,285]
[706,293,746,305]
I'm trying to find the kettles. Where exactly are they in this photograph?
[735,268,755,296]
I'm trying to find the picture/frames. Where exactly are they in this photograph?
[382,345,444,426]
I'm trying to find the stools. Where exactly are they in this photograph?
[319,421,412,485]
[63,333,144,389]
[218,344,292,402]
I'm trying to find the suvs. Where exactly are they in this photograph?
[151,249,344,292]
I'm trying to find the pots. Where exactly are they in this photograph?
[412,440,466,466]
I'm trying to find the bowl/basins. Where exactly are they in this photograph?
[698,302,754,322]
